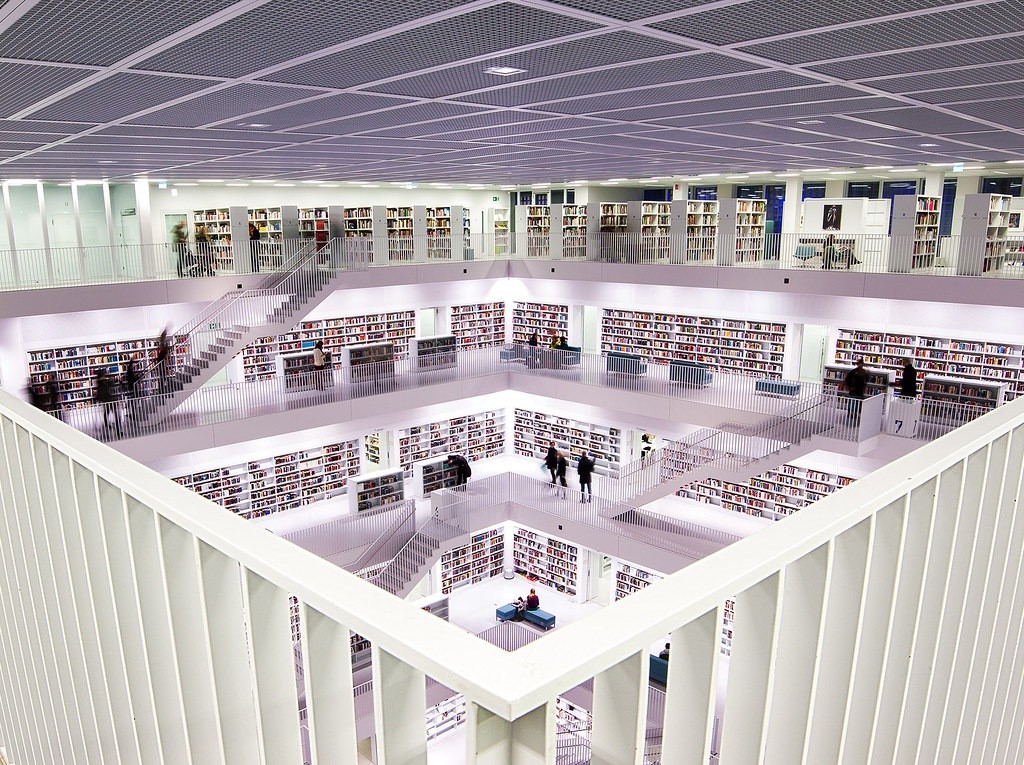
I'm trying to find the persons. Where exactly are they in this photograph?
[555,451,567,500]
[658,643,670,661]
[543,442,558,485]
[96,368,125,439]
[313,341,328,390]
[577,451,596,503]
[844,360,867,425]
[526,589,539,611]
[170,220,190,277]
[888,357,917,400]
[447,455,471,491]
[195,226,216,275]
[510,596,525,621]
[249,223,261,272]
[529,332,537,346]
[155,334,170,393]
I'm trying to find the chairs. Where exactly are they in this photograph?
[793,245,815,268]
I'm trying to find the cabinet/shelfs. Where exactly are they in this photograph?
[28,195,1024,742]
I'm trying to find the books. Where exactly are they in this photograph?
[289,530,735,656]
[194,199,1024,274]
[31,302,1023,426]
[170,410,857,524]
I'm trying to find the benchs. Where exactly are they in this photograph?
[649,653,668,683]
[562,345,581,363]
[755,380,800,401]
[607,352,646,377]
[496,601,555,630]
[500,348,530,362]
[669,361,712,389]
